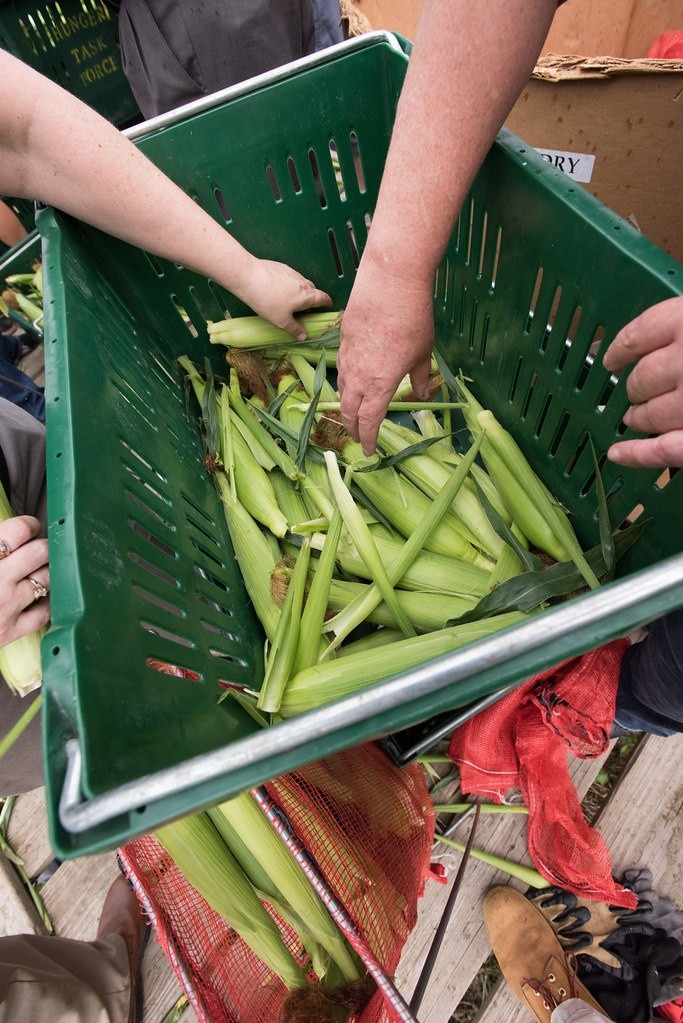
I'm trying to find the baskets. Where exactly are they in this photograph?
[0,0,143,126]
[36,32,683,869]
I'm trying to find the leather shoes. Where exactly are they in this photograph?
[96,874,151,1023]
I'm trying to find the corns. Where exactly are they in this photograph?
[0,484,48,697]
[1,259,44,328]
[149,309,586,1002]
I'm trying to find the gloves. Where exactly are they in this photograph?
[526,868,682,1020]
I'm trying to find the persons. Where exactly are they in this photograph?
[117,1,347,125]
[0,871,153,1023]
[336,0,683,1023]
[0,51,333,801]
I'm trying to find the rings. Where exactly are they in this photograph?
[26,577,47,600]
[0,540,10,559]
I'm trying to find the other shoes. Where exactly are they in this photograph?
[481,887,611,1023]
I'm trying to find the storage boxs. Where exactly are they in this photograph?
[0,229,44,359]
[377,683,527,769]
[338,0,683,346]
[34,30,683,860]
[0,0,143,233]
